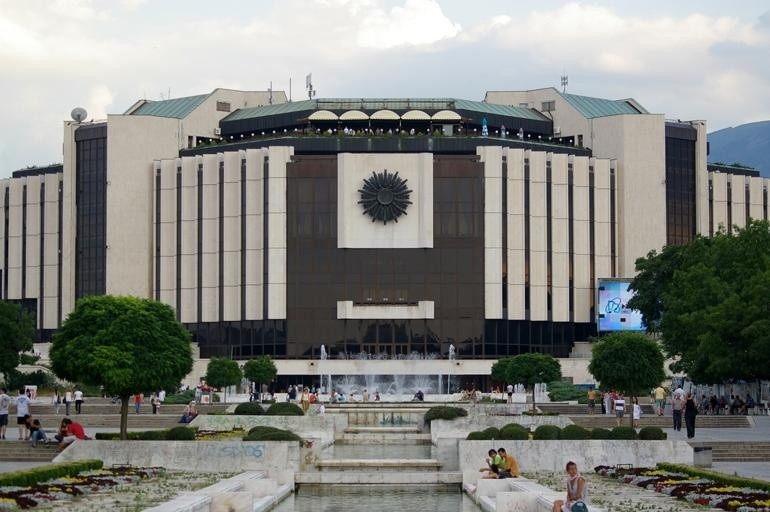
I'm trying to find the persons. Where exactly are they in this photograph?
[506,383,514,405]
[149,392,156,414]
[672,394,684,432]
[133,392,145,415]
[411,390,424,402]
[482,448,520,479]
[684,393,700,440]
[286,383,369,405]
[26,389,31,400]
[374,391,380,401]
[479,448,504,475]
[154,399,161,415]
[0,388,10,441]
[52,387,62,416]
[13,388,32,441]
[553,461,586,511]
[63,388,73,417]
[177,401,197,423]
[24,413,50,449]
[158,389,166,404]
[586,382,754,430]
[300,386,310,416]
[73,388,84,415]
[58,419,85,449]
[55,418,68,443]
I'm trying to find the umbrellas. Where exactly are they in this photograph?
[481,116,489,136]
